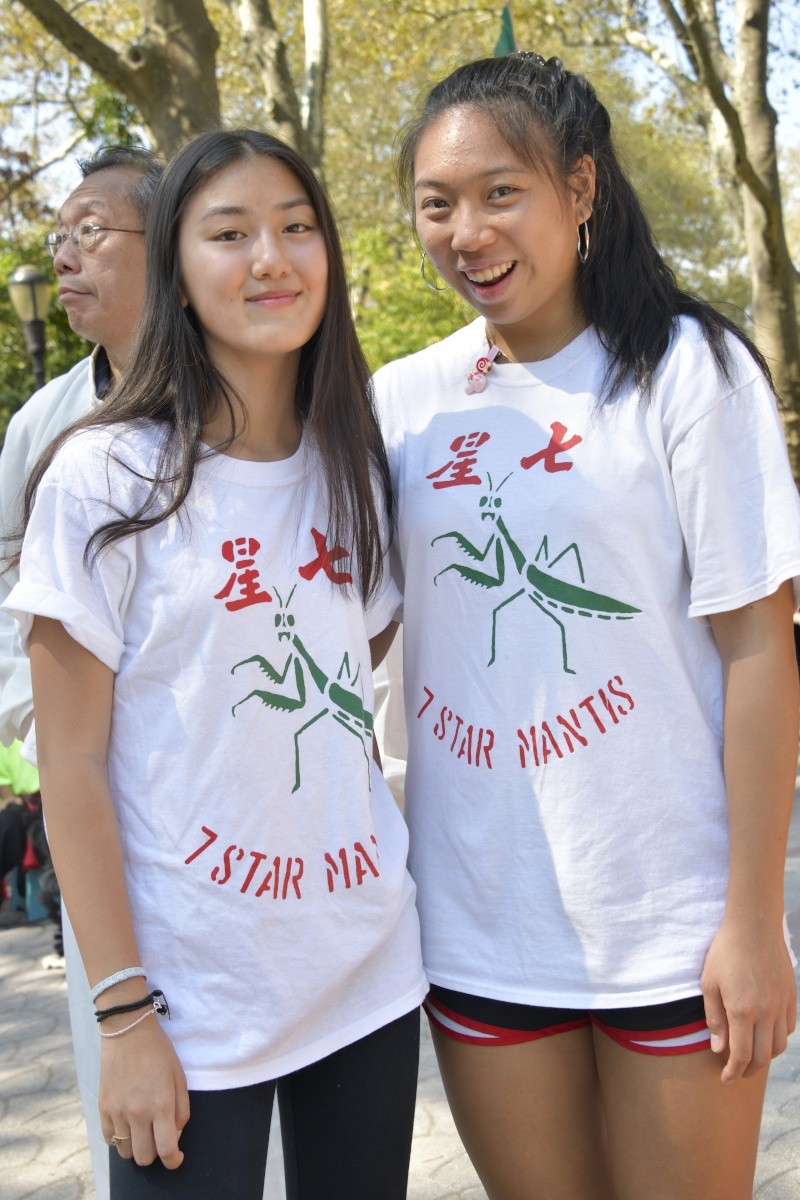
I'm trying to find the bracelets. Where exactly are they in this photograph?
[89,967,171,1038]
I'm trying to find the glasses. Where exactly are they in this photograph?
[44,223,146,257]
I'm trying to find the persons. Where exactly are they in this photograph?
[0,785,54,926]
[362,52,800,1200]
[0,145,408,1200]
[0,128,429,1200]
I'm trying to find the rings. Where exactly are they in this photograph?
[112,1136,132,1146]
